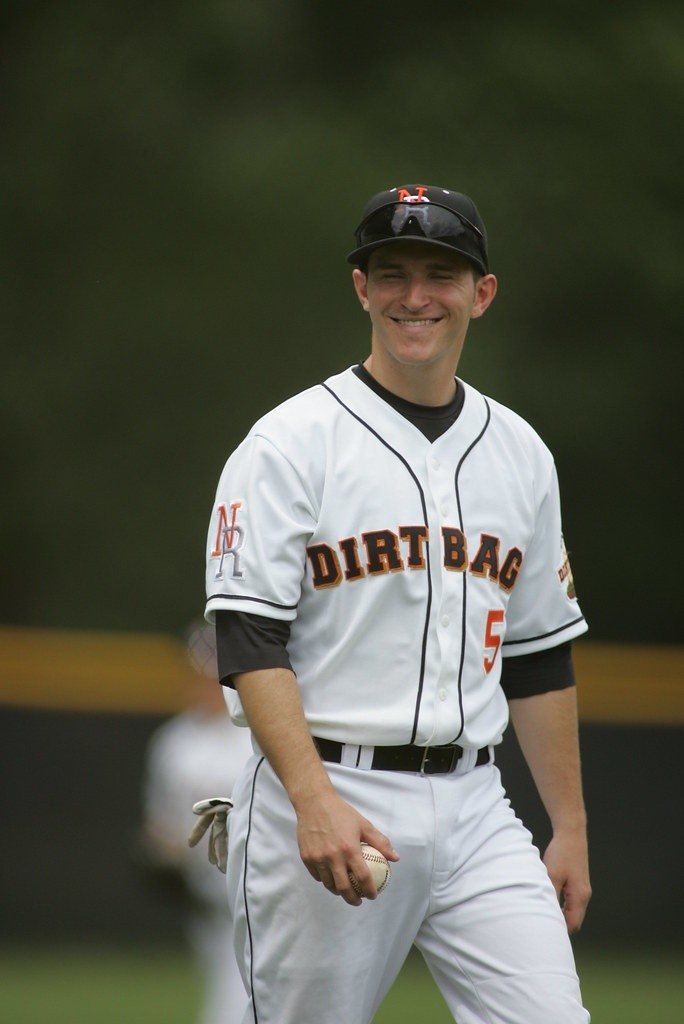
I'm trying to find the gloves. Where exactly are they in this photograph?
[188,797,233,874]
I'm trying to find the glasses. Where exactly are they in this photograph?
[355,201,490,274]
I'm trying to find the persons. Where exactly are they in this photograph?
[148,181,594,1024]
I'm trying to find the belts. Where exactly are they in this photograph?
[312,736,490,778]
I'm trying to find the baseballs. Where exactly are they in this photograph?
[345,840,391,899]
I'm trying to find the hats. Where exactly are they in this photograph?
[346,185,488,276]
